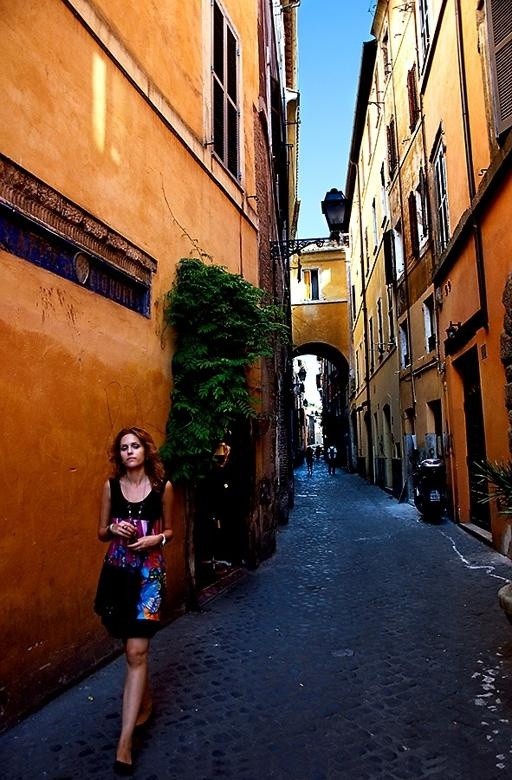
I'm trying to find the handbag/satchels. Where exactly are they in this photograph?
[93,559,140,621]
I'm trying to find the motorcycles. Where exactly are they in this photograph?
[409,447,449,526]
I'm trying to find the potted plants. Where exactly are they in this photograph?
[472,458,511,624]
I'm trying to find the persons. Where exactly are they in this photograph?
[304,446,314,475]
[326,443,337,476]
[316,444,322,458]
[89,426,176,777]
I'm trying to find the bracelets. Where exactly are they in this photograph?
[106,523,114,537]
[159,531,167,547]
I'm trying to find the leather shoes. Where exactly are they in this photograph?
[130,708,155,738]
[113,747,134,778]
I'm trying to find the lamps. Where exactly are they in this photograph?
[268,188,350,259]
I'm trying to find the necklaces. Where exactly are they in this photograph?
[123,474,147,531]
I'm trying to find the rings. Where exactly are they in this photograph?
[124,524,127,530]
